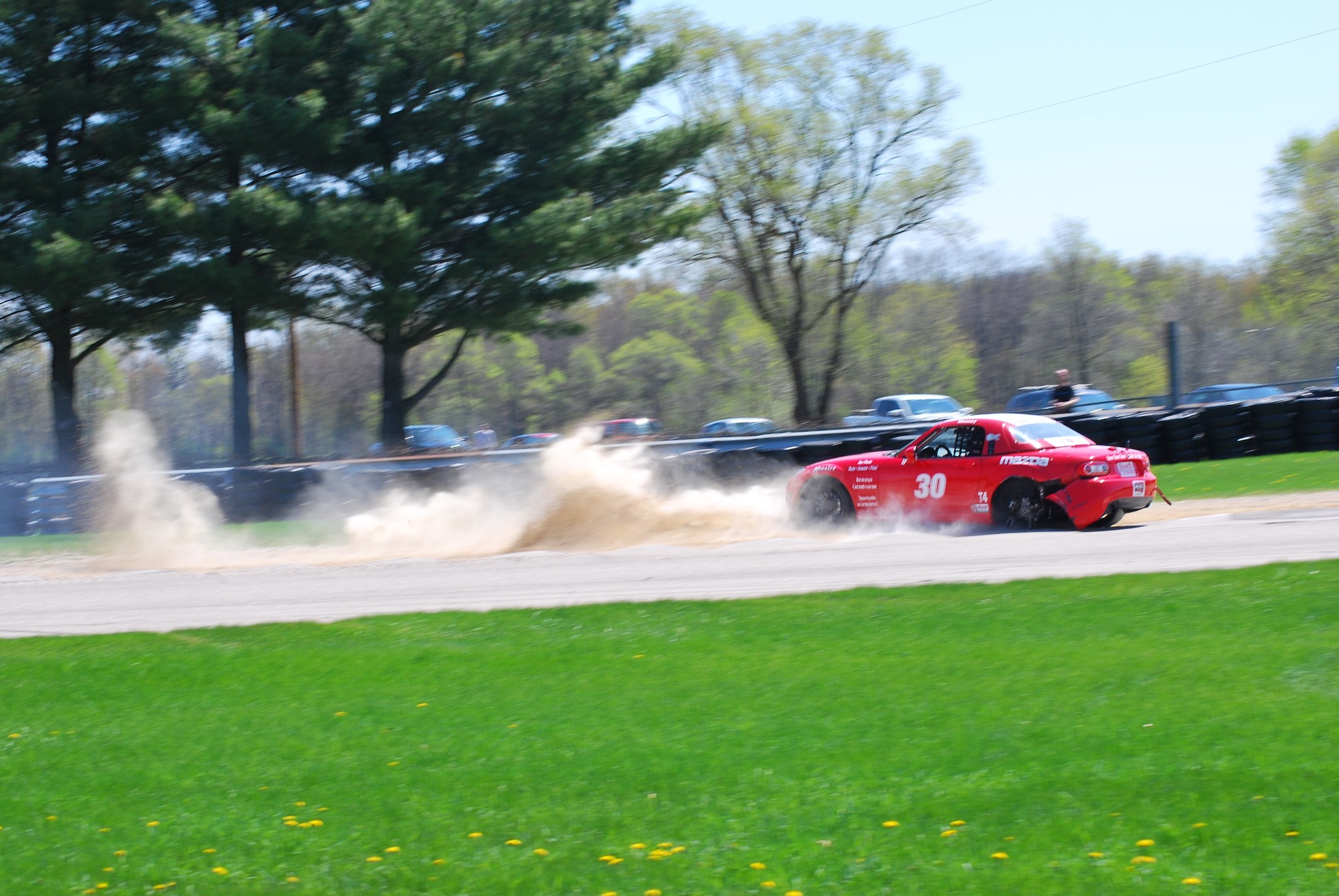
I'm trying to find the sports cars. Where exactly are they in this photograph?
[783,412,1172,533]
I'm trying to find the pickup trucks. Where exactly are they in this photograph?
[842,393,977,422]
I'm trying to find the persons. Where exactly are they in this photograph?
[1048,368,1081,415]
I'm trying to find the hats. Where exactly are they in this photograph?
[969,426,985,443]
[1054,368,1070,380]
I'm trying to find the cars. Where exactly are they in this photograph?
[701,417,785,433]
[1177,383,1285,403]
[366,424,465,452]
[504,431,562,447]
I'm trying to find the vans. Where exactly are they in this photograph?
[585,415,664,440]
[1004,388,1125,412]
[206,465,322,520]
